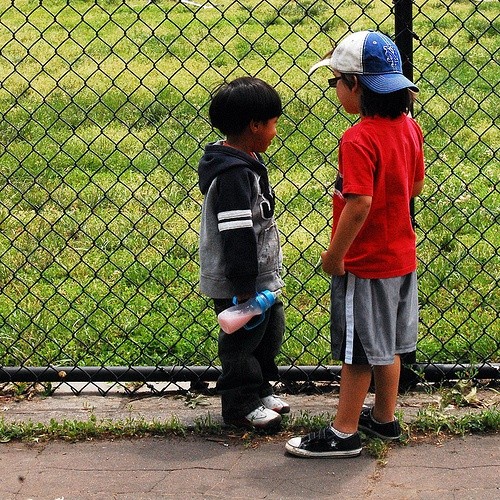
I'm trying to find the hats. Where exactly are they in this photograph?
[307,28,420,94]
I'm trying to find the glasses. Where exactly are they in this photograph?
[327,77,342,88]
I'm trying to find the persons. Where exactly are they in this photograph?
[198,77,290,435]
[285,28,425,460]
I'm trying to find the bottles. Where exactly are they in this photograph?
[217,289,282,334]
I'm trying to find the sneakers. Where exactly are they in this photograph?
[224,405,283,432]
[284,421,364,460]
[259,396,291,414]
[356,404,402,443]
